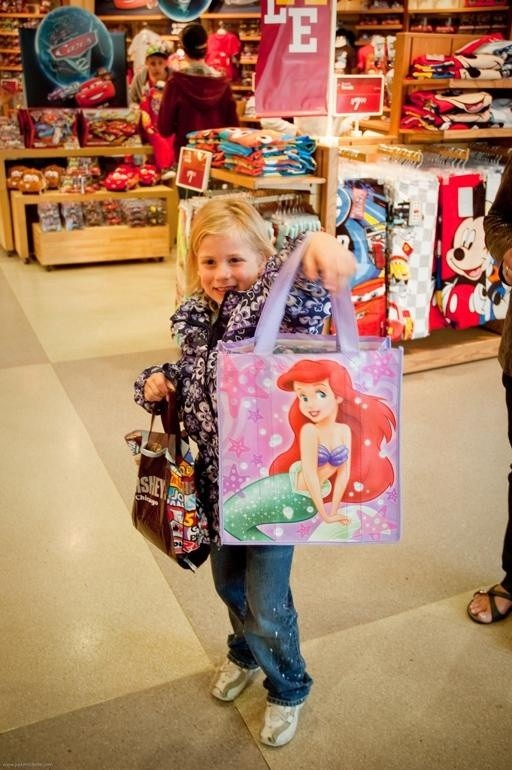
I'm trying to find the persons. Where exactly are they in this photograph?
[262,357,394,527]
[464,147,512,626]
[127,45,174,111]
[156,23,239,165]
[134,198,360,748]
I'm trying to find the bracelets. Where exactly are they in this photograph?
[505,264,511,279]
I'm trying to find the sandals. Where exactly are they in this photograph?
[467,584,512,625]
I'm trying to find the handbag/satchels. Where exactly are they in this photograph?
[214,334,403,547]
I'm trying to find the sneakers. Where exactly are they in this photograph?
[259,700,306,748]
[208,658,261,703]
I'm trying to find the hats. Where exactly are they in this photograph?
[144,43,168,60]
[179,24,208,49]
